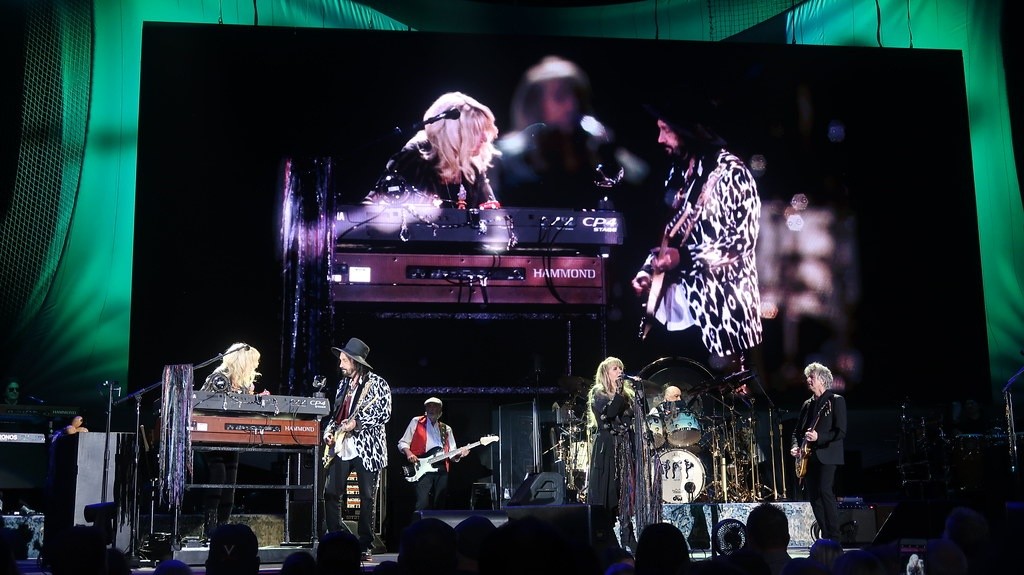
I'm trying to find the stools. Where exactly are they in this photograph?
[470,482,497,511]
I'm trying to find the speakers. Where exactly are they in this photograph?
[833,505,878,547]
[0,433,52,489]
[505,472,563,506]
[410,504,591,548]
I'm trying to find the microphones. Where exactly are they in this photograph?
[622,374,643,381]
[244,345,250,351]
[430,107,461,124]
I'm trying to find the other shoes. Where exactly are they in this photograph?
[361,548,373,561]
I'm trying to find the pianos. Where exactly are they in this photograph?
[147,390,332,548]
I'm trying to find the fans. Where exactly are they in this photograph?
[712,519,747,561]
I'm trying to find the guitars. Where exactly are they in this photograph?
[321,398,366,470]
[795,399,833,479]
[401,433,500,485]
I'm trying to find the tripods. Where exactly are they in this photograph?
[704,380,781,502]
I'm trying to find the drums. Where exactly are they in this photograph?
[664,407,702,448]
[642,414,665,450]
[569,440,593,473]
[649,448,707,504]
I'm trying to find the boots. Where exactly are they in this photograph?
[217,509,231,524]
[204,506,218,535]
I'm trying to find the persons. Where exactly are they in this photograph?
[907,554,924,575]
[0,505,1024,575]
[632,98,763,369]
[0,381,32,405]
[497,59,614,207]
[323,339,393,561]
[398,398,470,510]
[953,399,988,477]
[651,386,694,537]
[201,343,270,530]
[791,362,847,547]
[586,356,650,545]
[366,92,500,209]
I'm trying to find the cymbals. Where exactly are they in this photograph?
[709,416,723,420]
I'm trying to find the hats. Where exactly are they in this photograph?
[424,397,442,408]
[330,338,372,369]
[208,523,258,574]
